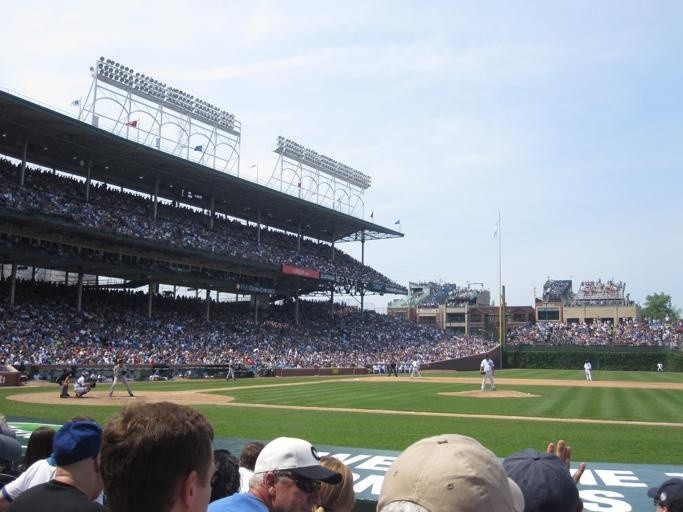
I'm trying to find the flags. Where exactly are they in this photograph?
[194,146,202,151]
[72,99,80,106]
[126,120,138,126]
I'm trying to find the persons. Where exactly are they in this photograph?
[584,360,592,383]
[504,314,683,350]
[0,157,497,400]
[543,279,625,305]
[0,399,586,511]
[657,362,663,372]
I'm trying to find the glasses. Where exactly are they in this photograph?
[275,473,322,492]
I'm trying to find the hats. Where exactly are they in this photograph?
[0,433,22,464]
[375,433,525,512]
[51,420,103,465]
[253,436,343,484]
[502,448,579,512]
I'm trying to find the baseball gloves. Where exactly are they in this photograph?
[480,371,486,375]
[90,382,96,388]
